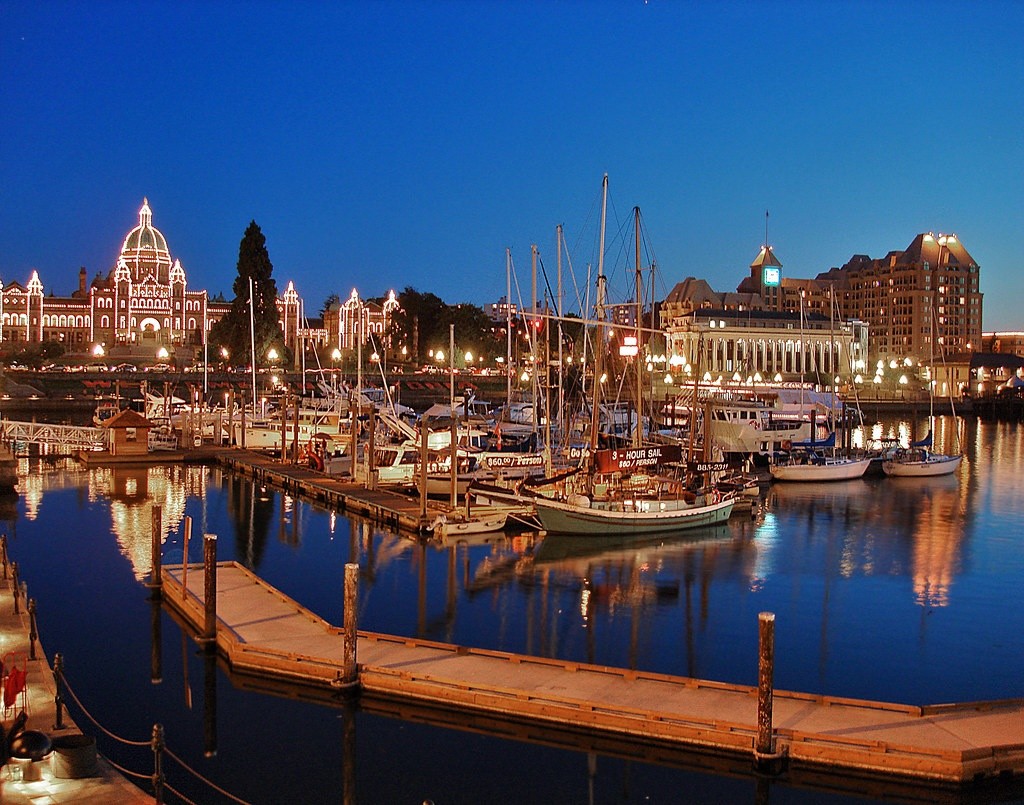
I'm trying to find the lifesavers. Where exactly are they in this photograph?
[710,489,721,505]
[749,420,758,430]
[429,461,439,471]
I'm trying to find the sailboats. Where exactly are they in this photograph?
[92,172,963,534]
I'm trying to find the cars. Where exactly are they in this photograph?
[422,364,445,373]
[39,362,286,374]
